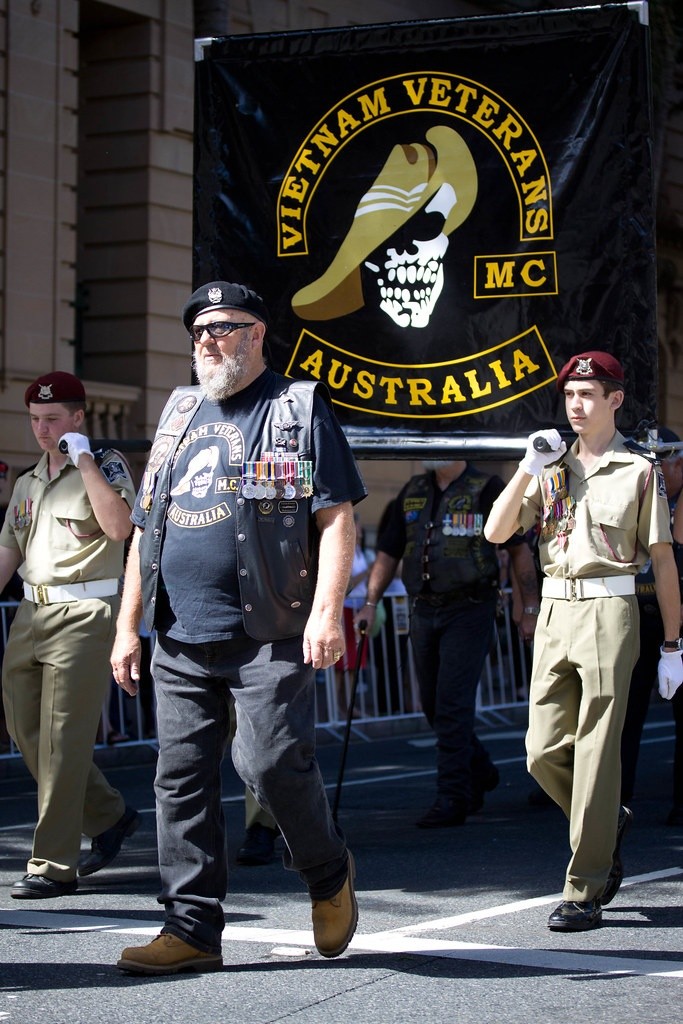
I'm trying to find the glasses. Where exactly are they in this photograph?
[189,321,256,341]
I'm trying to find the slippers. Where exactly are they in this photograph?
[97,731,129,743]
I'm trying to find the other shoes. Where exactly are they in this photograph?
[527,787,552,804]
[666,806,683,825]
[235,821,276,864]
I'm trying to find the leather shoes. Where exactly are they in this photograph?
[467,764,501,816]
[78,805,144,876]
[599,806,633,905]
[547,896,602,929]
[415,796,469,828]
[10,873,79,899]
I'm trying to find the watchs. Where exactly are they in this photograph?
[523,606,540,615]
[663,638,683,650]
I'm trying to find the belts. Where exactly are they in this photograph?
[23,578,118,606]
[541,575,635,601]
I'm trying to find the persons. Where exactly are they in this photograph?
[227,695,288,865]
[483,350,683,929]
[323,427,683,828]
[110,282,368,973]
[0,370,141,898]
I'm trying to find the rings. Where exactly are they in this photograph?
[334,651,341,661]
[525,636,529,640]
[354,623,358,629]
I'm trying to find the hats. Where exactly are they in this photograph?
[182,281,271,338]
[25,371,86,408]
[556,351,625,394]
[657,427,681,458]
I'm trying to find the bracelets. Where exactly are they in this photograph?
[366,601,377,607]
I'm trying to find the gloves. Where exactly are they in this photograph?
[658,645,683,700]
[57,432,95,468]
[519,428,567,476]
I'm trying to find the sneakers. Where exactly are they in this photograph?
[116,932,223,976]
[311,848,359,958]
